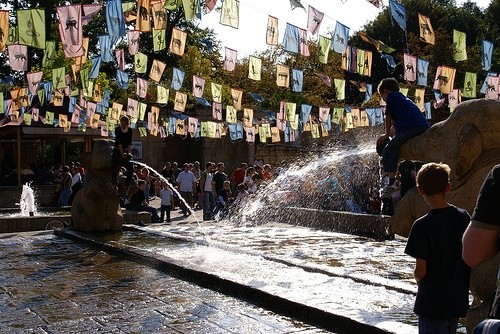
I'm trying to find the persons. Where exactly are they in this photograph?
[460,163,500,269]
[55,161,416,222]
[111,116,135,190]
[0,165,55,185]
[403,161,473,334]
[377,77,430,198]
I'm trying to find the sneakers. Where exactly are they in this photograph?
[381,181,399,197]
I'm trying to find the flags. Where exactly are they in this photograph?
[0,0,500,144]
